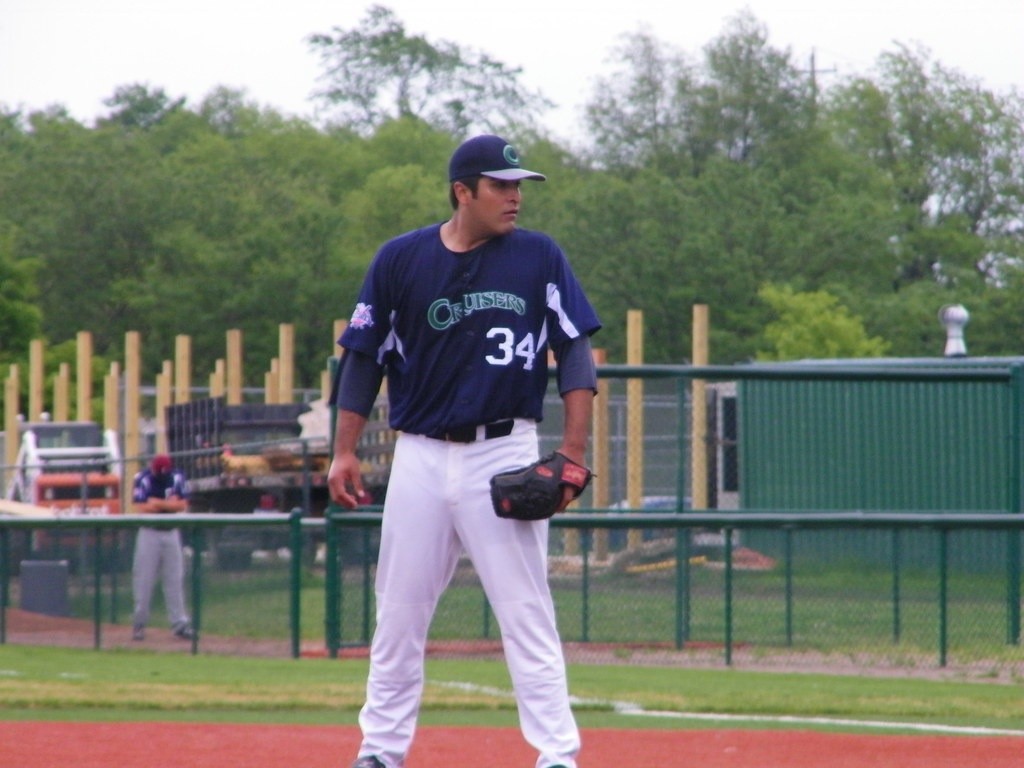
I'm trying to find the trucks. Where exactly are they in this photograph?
[8,421,133,576]
[163,394,397,570]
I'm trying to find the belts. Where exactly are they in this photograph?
[425,419,517,443]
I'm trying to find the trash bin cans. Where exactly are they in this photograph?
[18,559,73,617]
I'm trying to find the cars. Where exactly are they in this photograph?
[584,496,691,551]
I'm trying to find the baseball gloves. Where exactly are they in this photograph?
[490,450,597,521]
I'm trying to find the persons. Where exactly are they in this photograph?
[131,454,200,641]
[326,135,603,768]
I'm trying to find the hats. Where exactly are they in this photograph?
[151,454,174,475]
[448,135,547,184]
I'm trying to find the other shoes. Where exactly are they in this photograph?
[132,624,145,640]
[175,626,197,639]
